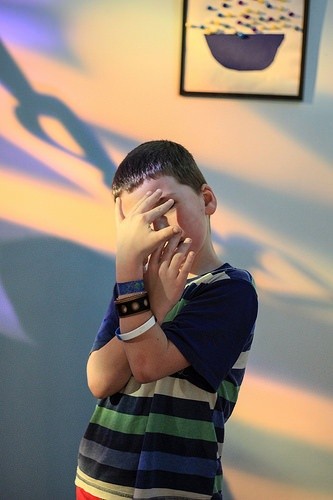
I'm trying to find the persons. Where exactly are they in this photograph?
[70,139,258,499]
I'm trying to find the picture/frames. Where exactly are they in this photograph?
[178,0,312,102]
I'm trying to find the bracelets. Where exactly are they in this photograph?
[115,279,145,296]
[112,291,152,320]
[115,314,158,342]
